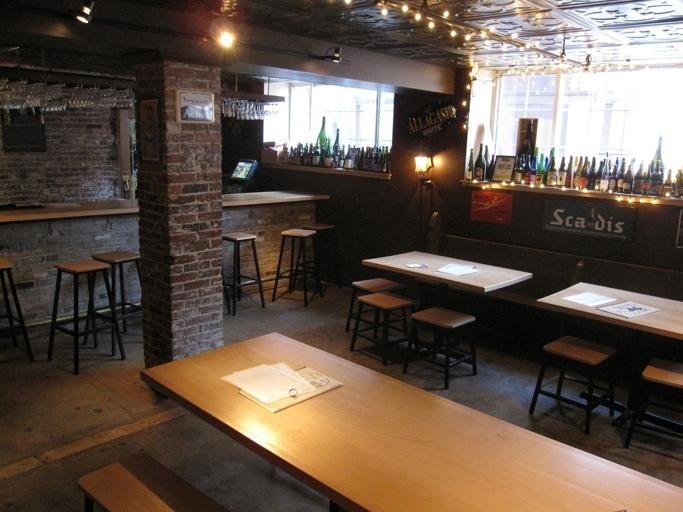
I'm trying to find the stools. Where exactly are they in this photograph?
[272,229,323,307]
[407,305,478,389]
[83,253,146,344]
[623,355,681,451]
[220,232,265,314]
[350,294,410,364]
[295,223,335,296]
[77,462,176,511]
[0,259,36,365]
[46,259,127,373]
[342,277,402,338]
[530,334,615,434]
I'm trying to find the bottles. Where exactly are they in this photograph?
[581,156,589,189]
[651,160,655,174]
[622,164,633,193]
[340,144,392,174]
[546,157,557,186]
[530,155,537,183]
[524,155,531,185]
[474,143,485,181]
[565,155,573,188]
[609,159,619,192]
[535,153,544,186]
[489,153,495,181]
[557,156,566,186]
[617,158,626,192]
[652,160,663,196]
[514,158,523,184]
[662,169,673,196]
[594,161,603,191]
[314,116,339,167]
[543,147,555,184]
[633,163,644,195]
[574,156,583,188]
[484,145,489,180]
[280,142,313,167]
[609,160,612,177]
[675,168,683,197]
[599,151,609,191]
[466,148,474,180]
[642,165,652,195]
[574,156,579,173]
[588,156,597,189]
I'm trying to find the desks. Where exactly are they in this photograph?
[360,251,533,365]
[533,281,682,435]
[141,332,683,512]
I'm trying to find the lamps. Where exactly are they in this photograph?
[415,153,433,187]
[77,2,93,23]
[324,45,340,64]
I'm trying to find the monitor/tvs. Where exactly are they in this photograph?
[229,159,259,182]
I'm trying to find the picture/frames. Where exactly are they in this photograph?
[176,89,216,124]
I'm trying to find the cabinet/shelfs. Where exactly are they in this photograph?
[461,176,683,301]
[262,164,392,285]
